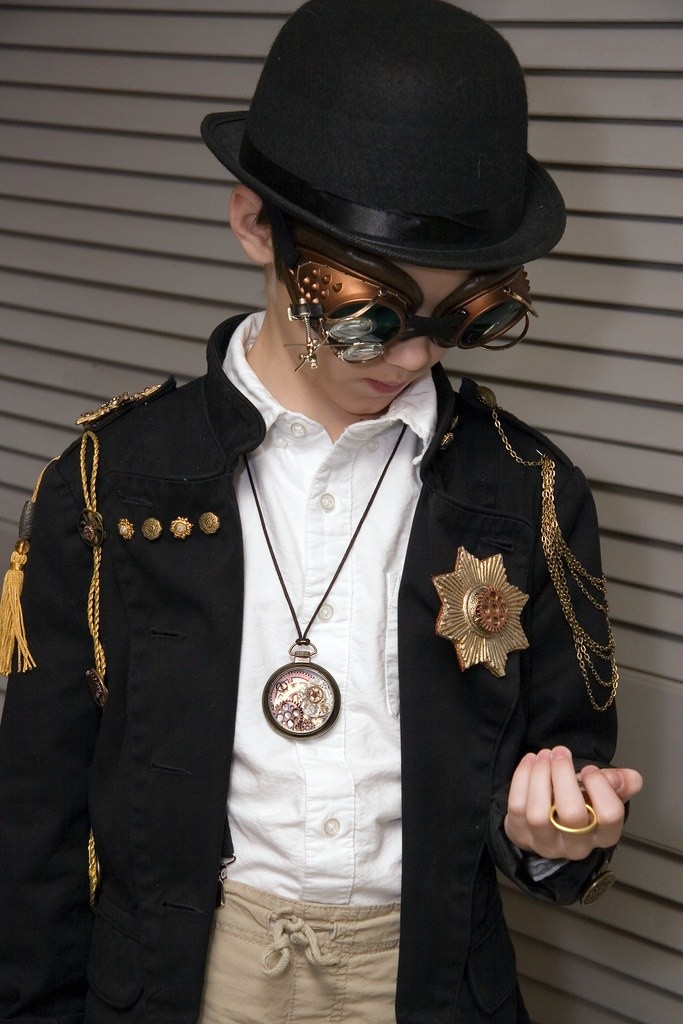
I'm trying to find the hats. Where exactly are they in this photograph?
[200,0,566,270]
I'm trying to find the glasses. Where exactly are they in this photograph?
[271,220,537,373]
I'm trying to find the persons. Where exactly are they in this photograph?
[0,0,642,1024]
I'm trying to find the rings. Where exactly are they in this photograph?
[548,804,597,834]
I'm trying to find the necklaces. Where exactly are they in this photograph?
[239,423,408,738]
